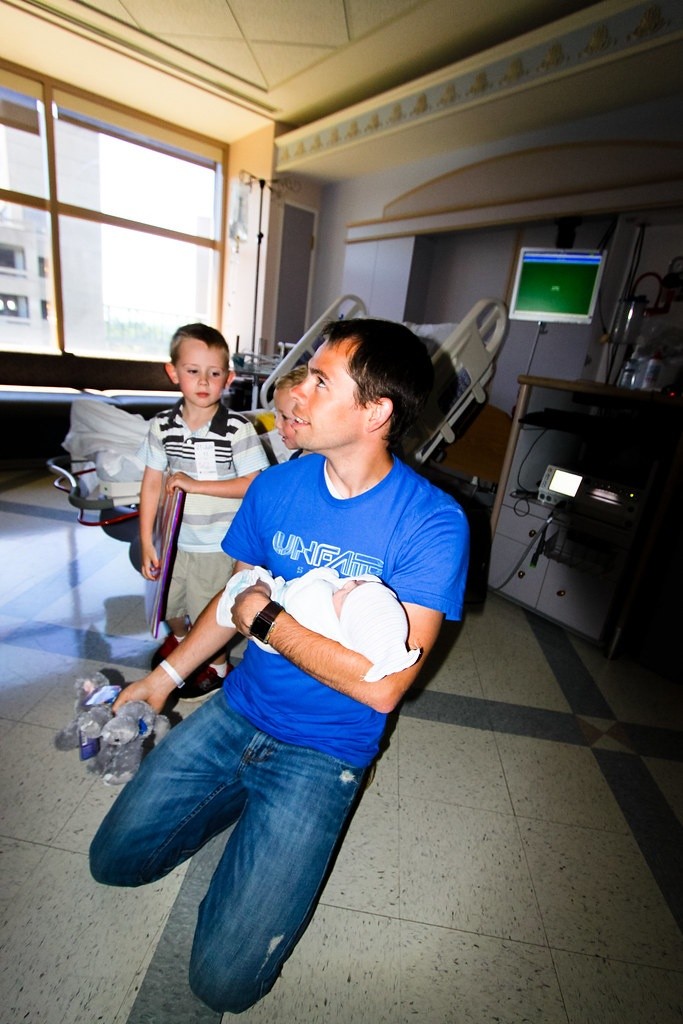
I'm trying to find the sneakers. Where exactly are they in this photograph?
[174,663,235,703]
[150,631,179,672]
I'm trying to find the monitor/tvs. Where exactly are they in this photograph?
[506,247,608,326]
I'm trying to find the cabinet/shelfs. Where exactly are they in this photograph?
[493,375,682,674]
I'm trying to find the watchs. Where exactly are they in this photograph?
[247,600,285,644]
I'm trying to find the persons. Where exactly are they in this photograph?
[138,322,273,698]
[83,318,470,1015]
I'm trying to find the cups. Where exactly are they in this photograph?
[619,348,664,394]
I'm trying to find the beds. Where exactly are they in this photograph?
[44,293,519,582]
